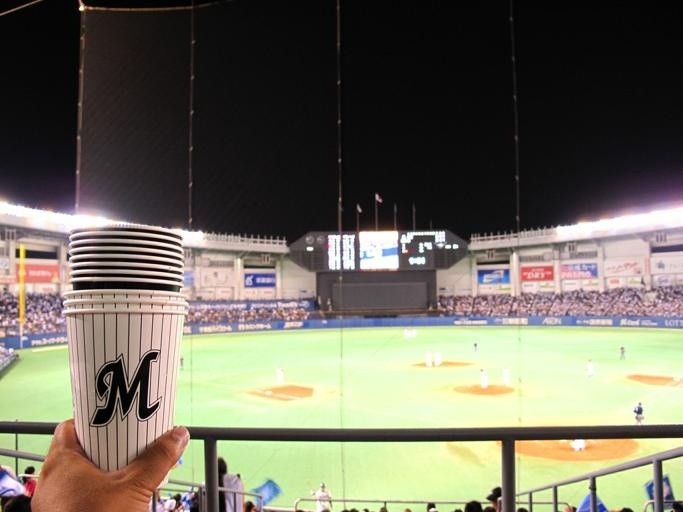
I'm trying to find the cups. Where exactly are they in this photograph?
[62,223,188,485]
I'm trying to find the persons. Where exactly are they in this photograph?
[631,400,645,425]
[437,285,683,319]
[2,287,66,335]
[478,367,490,390]
[618,344,627,360]
[311,483,683,512]
[28,416,189,512]
[150,458,254,512]
[1,465,38,511]
[184,305,311,325]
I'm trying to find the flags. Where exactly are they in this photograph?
[355,203,363,214]
[374,193,383,205]
